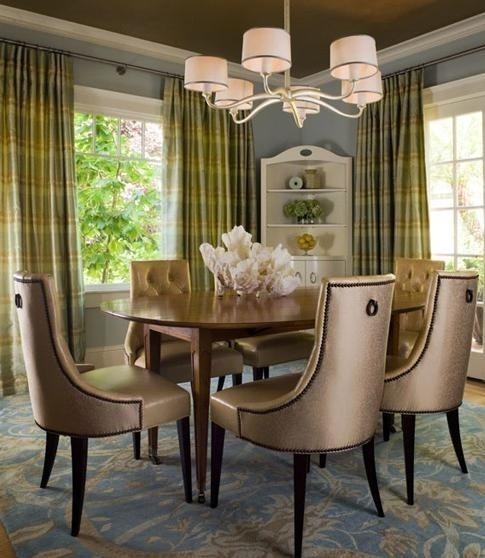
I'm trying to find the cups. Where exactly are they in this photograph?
[296,208,305,225]
[316,207,326,224]
[307,207,320,223]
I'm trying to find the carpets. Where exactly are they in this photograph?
[0,341,485,558]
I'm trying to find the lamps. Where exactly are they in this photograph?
[182,26,384,128]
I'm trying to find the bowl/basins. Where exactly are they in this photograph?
[297,240,317,256]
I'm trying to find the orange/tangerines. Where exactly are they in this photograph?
[295,233,317,249]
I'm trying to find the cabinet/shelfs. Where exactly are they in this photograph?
[260,144,354,286]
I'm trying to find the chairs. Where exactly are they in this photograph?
[378,268,480,505]
[205,271,396,557]
[239,328,318,379]
[8,269,193,537]
[123,259,244,388]
[392,258,446,353]
